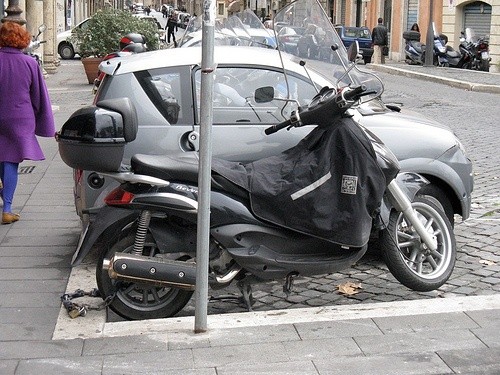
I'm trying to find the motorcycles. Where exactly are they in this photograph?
[55,0,457,321]
[440,31,477,68]
[403,22,449,67]
[21,24,46,65]
[466,28,491,72]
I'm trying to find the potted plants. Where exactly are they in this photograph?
[66,3,161,85]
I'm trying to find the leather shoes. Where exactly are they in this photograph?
[1,211,21,224]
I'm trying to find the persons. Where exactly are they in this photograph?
[145,5,151,15]
[410,23,420,33]
[161,5,168,19]
[0,20,56,224]
[371,17,388,64]
[164,14,179,43]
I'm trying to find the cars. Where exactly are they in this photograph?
[176,27,276,47]
[73,45,473,263]
[278,25,304,53]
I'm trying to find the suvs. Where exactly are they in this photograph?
[298,24,333,59]
[56,14,165,59]
[317,25,374,64]
